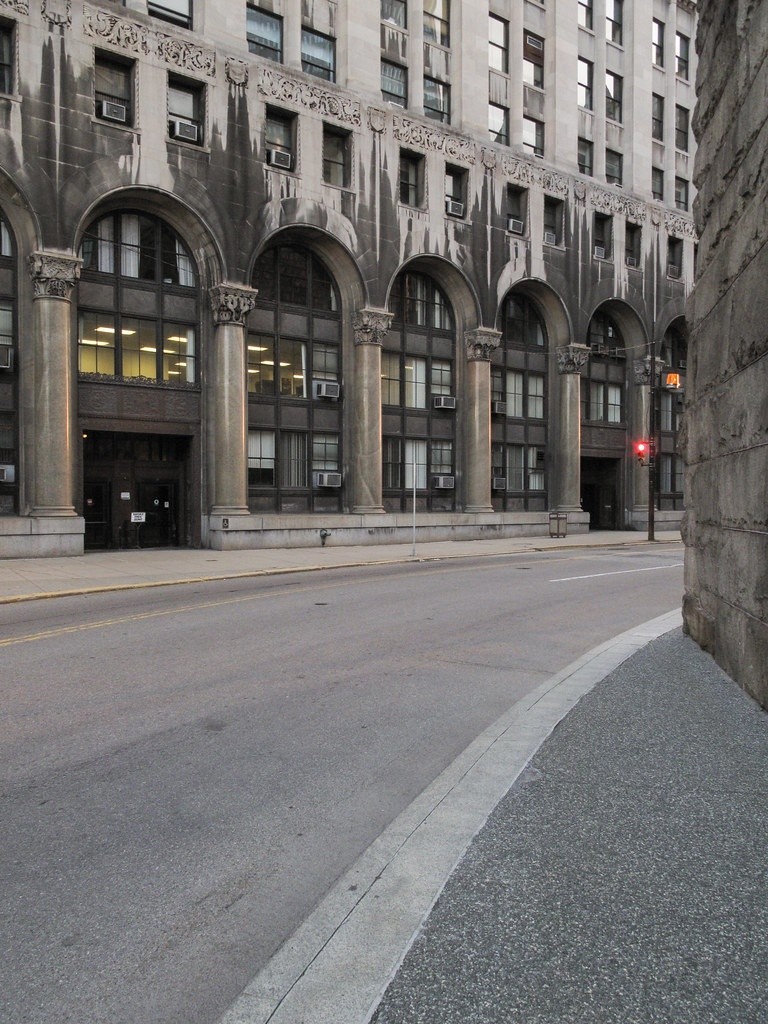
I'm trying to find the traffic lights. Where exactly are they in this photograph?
[634,441,647,460]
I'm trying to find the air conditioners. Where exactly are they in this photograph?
[434,397,455,409]
[316,473,342,488]
[446,201,463,218]
[491,401,506,414]
[169,121,198,145]
[491,477,505,490]
[595,246,605,259]
[267,149,291,171]
[317,383,340,398]
[98,100,126,125]
[507,219,523,236]
[435,476,455,489]
[545,232,556,246]
